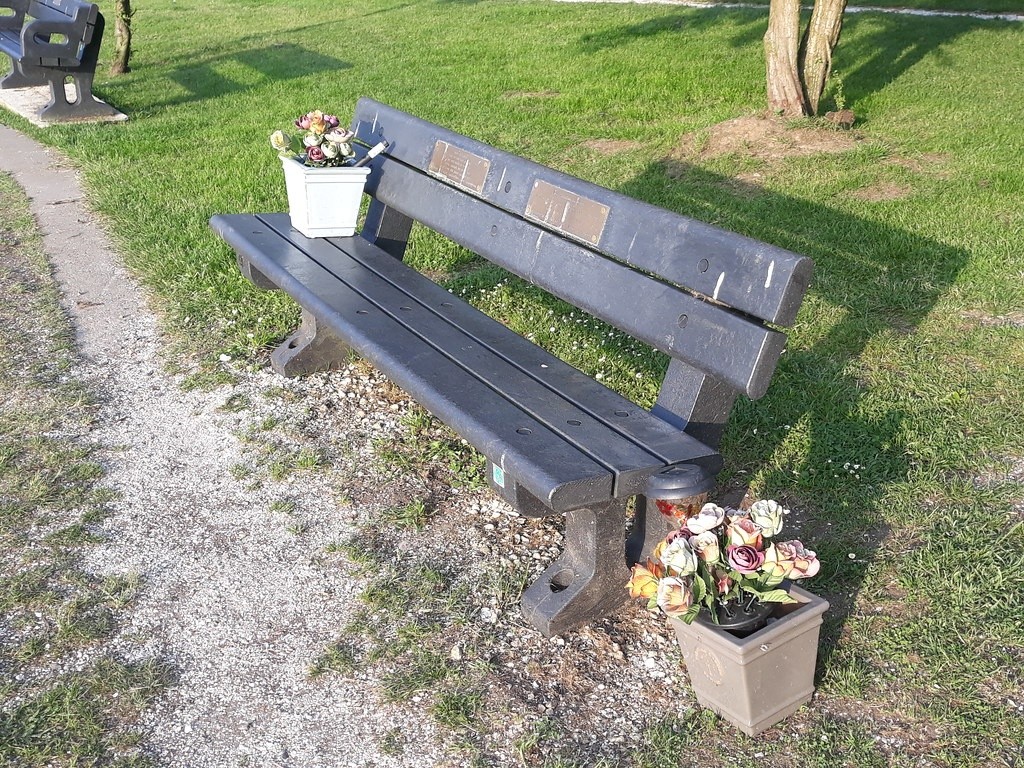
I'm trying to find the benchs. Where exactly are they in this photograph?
[0,0,115,122]
[206,96,818,625]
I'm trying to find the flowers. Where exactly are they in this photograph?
[624,499,822,625]
[270,109,356,167]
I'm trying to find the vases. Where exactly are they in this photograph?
[279,153,372,240]
[668,584,830,739]
